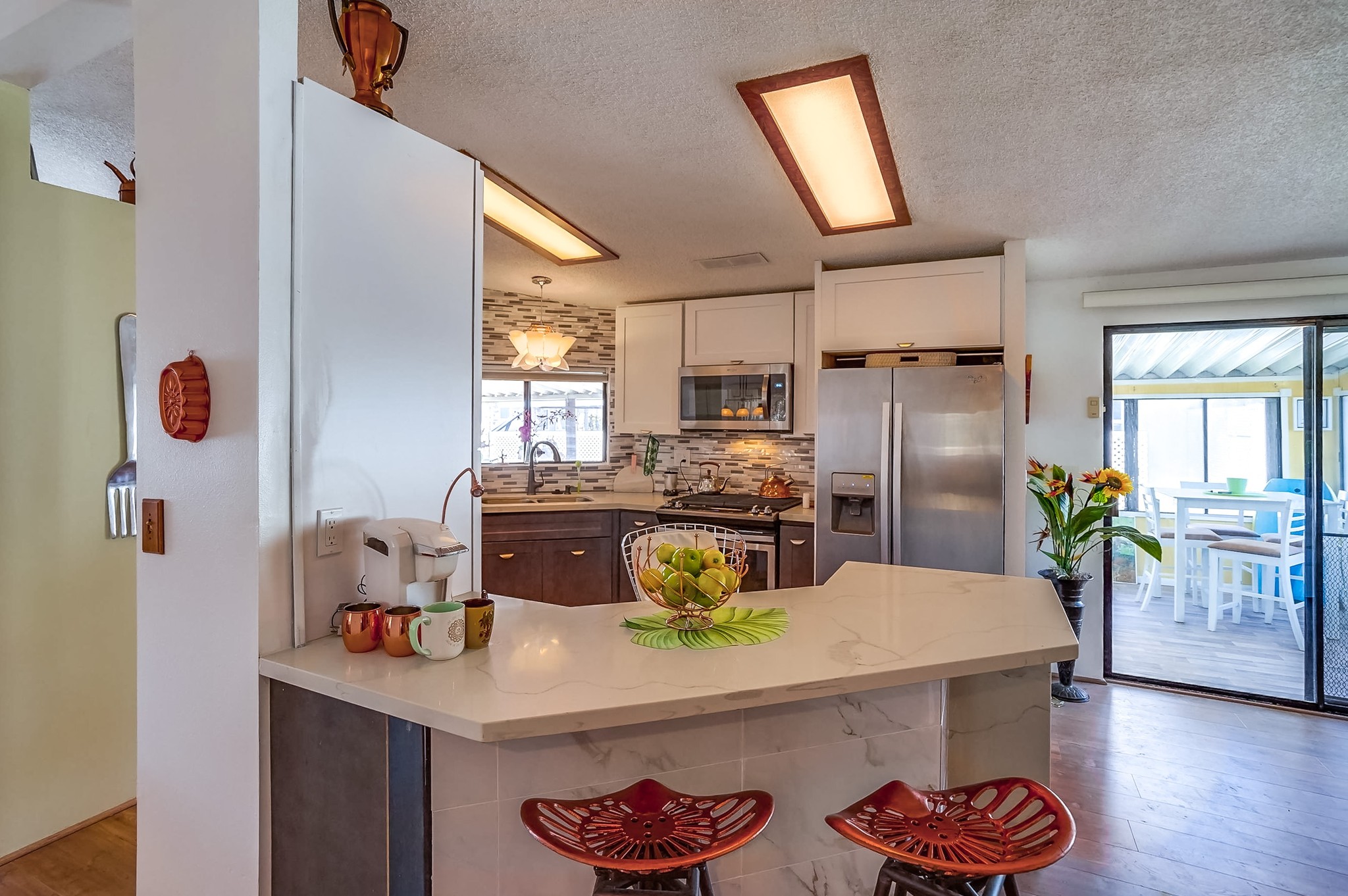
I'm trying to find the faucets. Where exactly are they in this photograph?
[526,440,561,495]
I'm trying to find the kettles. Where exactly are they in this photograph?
[758,461,795,499]
[697,461,730,495]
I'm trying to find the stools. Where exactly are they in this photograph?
[521,779,775,896]
[823,777,1077,896]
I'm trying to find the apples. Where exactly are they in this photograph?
[639,544,739,608]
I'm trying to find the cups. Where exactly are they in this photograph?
[1227,478,1248,494]
[460,597,495,649]
[409,601,466,661]
[664,471,678,491]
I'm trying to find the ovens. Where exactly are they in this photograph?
[659,522,777,594]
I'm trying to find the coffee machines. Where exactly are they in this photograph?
[364,518,469,606]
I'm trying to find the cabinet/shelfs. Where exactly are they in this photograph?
[614,290,815,438]
[482,510,814,607]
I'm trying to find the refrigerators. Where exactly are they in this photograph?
[813,364,1007,586]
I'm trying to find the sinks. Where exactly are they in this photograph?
[534,494,595,503]
[481,496,538,504]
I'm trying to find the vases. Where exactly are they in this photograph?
[1037,569,1094,702]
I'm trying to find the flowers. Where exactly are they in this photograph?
[1026,455,1162,581]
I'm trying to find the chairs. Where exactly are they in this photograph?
[1137,484,1348,652]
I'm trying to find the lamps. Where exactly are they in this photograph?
[507,276,577,372]
[735,54,912,237]
[457,149,619,267]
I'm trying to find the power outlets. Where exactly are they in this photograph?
[318,507,343,557]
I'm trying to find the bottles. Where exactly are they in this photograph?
[342,601,384,653]
[382,604,421,657]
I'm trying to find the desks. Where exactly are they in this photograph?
[1157,488,1342,624]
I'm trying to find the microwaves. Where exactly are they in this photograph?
[678,363,794,435]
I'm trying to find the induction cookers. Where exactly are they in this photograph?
[656,493,803,531]
